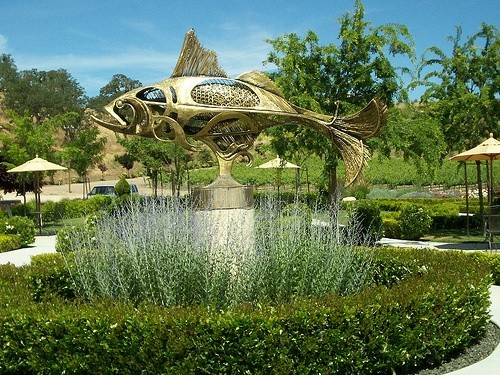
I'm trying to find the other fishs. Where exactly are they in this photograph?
[89,27,391,190]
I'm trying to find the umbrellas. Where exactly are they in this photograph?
[5,154,68,238]
[252,155,302,220]
[449,133,500,244]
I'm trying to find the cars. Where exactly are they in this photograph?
[86,184,138,197]
